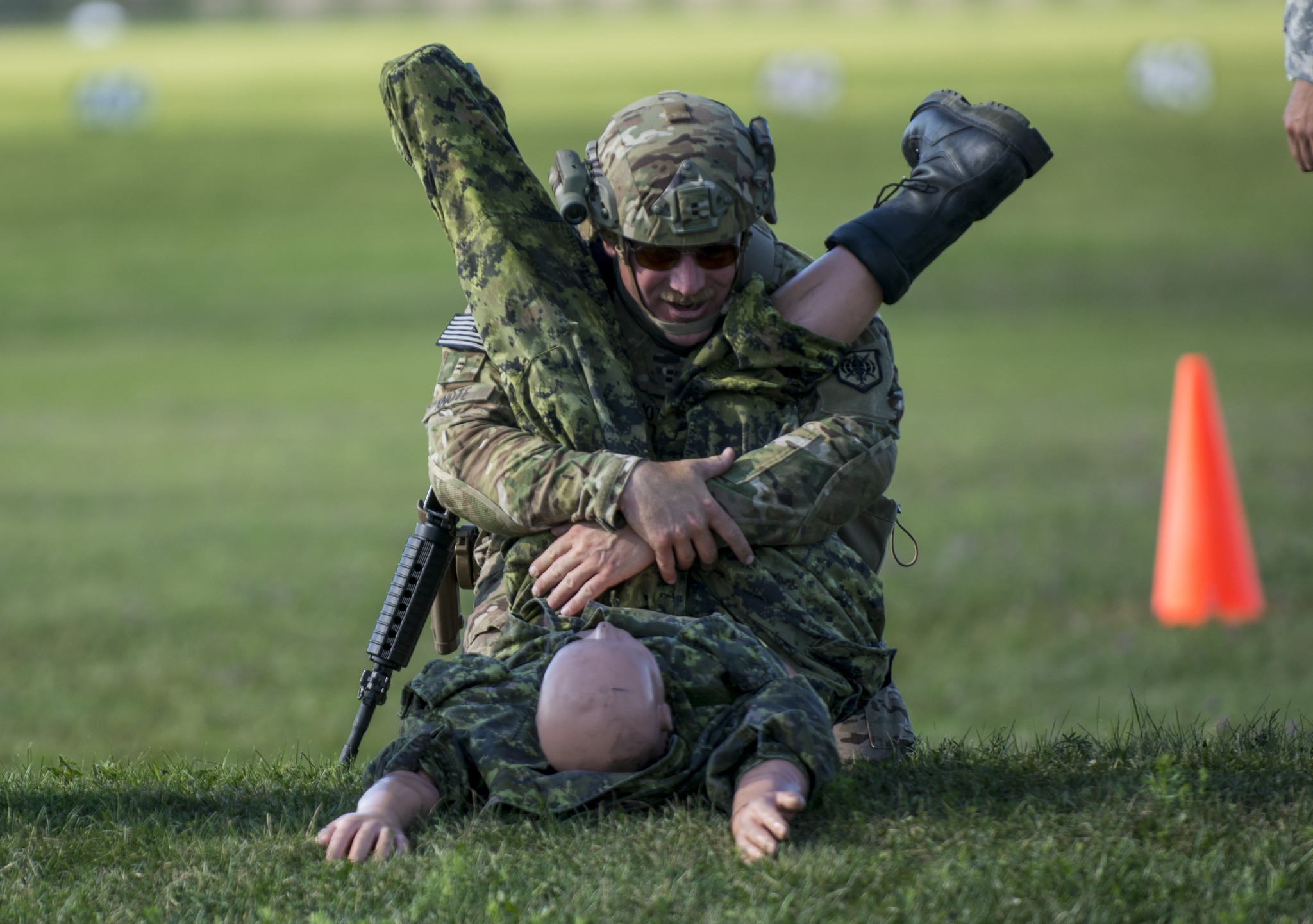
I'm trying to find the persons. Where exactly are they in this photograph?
[416,89,925,768]
[309,38,1056,877]
[1281,0,1313,178]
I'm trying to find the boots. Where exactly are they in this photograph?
[824,89,1054,305]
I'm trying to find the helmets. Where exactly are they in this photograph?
[587,90,765,246]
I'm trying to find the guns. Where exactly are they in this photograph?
[328,479,482,787]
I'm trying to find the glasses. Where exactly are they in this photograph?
[619,225,754,271]
